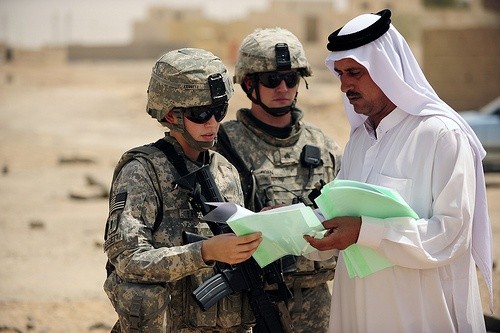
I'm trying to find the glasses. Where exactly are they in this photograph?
[182,103,228,123]
[247,71,300,88]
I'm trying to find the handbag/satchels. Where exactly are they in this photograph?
[115,281,172,333]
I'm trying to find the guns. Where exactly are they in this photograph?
[187,157,300,332]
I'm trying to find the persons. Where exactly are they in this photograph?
[259,7,492,333]
[103,46,260,332]
[210,26,344,332]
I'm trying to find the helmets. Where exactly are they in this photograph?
[146,47,234,121]
[232,27,313,84]
